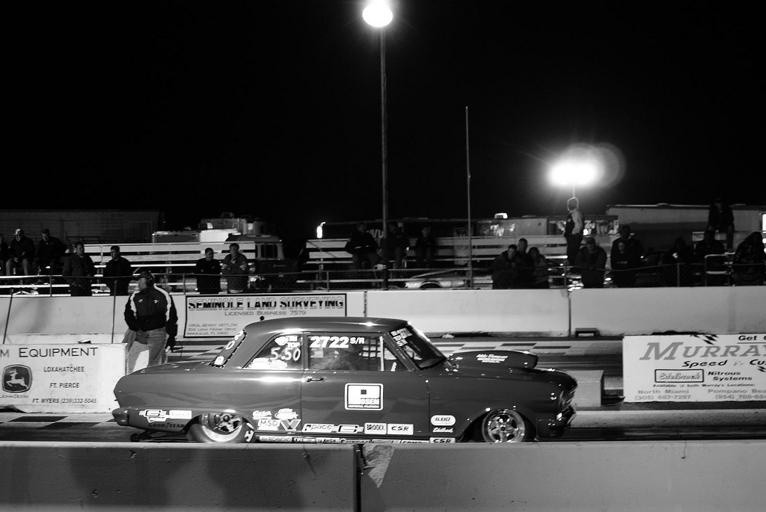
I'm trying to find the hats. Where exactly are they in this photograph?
[15,228,25,236]
[134,271,153,279]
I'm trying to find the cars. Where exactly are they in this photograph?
[404,271,494,290]
[112,316,577,443]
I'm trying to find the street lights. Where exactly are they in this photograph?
[362,0,394,238]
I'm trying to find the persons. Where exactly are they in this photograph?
[489,192,765,289]
[192,246,223,295]
[32,229,66,287]
[220,242,249,294]
[122,270,178,377]
[3,227,38,295]
[345,220,380,281]
[415,220,437,268]
[58,241,97,297]
[100,245,133,296]
[378,220,410,269]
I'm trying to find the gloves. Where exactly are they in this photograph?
[165,336,177,353]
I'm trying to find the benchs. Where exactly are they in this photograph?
[69,235,567,284]
